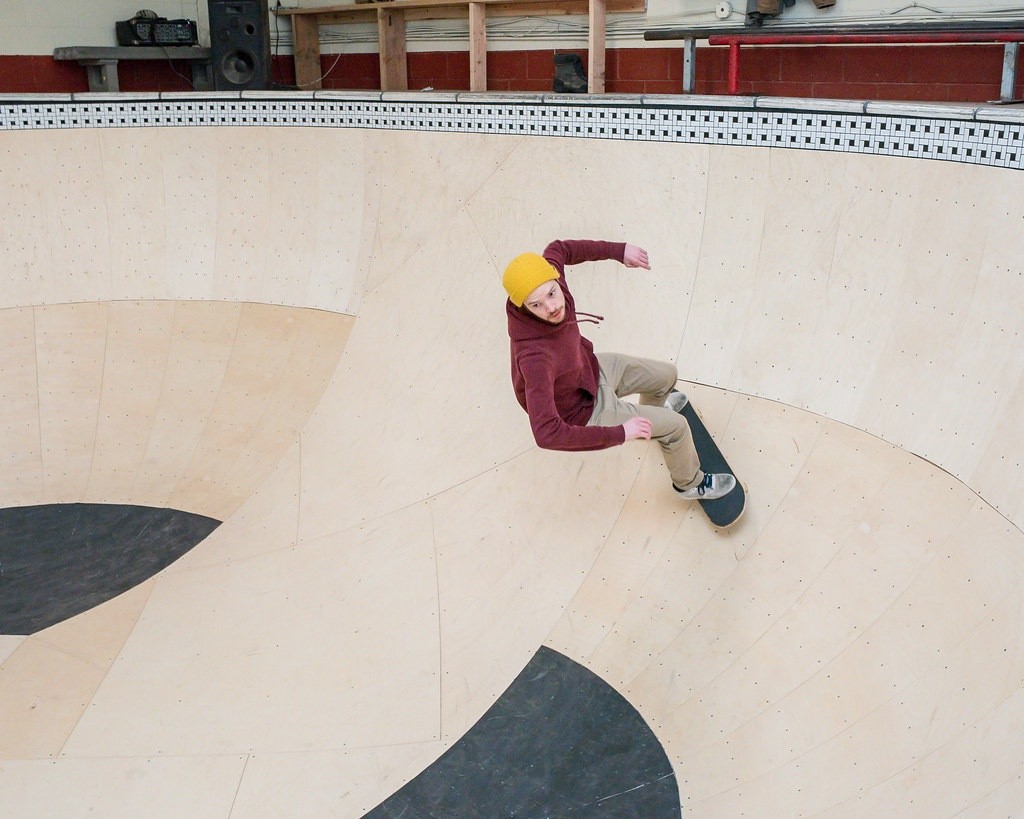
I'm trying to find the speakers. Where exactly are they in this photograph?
[208,0,271,90]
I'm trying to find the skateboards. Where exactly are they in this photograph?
[670,388,746,529]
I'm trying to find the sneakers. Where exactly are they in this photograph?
[671,473,737,499]
[665,392,688,412]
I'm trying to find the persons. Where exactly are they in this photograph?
[502,239,737,499]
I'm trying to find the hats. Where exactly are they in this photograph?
[502,252,560,308]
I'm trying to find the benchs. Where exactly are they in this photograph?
[52,46,215,91]
